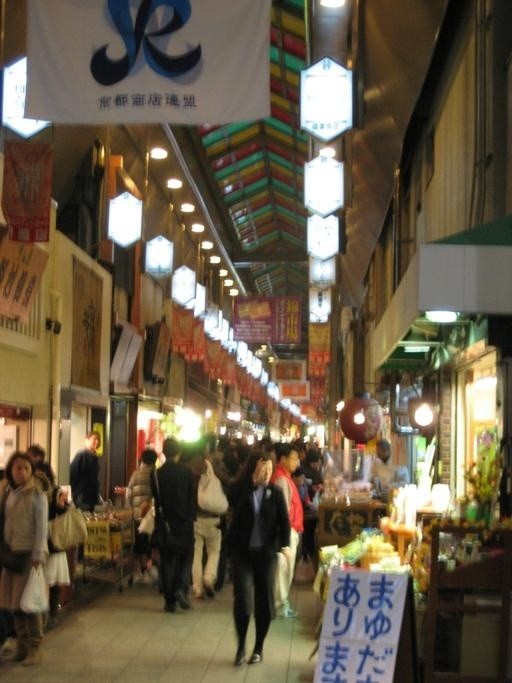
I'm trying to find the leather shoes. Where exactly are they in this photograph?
[248,653,262,663]
[235,649,245,666]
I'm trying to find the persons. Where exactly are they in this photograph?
[69,432,102,562]
[127,431,336,617]
[370,440,402,487]
[0,446,71,666]
[480,430,495,452]
[227,453,291,666]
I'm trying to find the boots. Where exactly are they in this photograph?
[4,613,43,666]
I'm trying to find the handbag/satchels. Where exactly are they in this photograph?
[197,473,229,515]
[46,508,88,552]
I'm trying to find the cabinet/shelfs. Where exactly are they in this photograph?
[81,509,136,593]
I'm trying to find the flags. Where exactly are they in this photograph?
[25,0,271,122]
